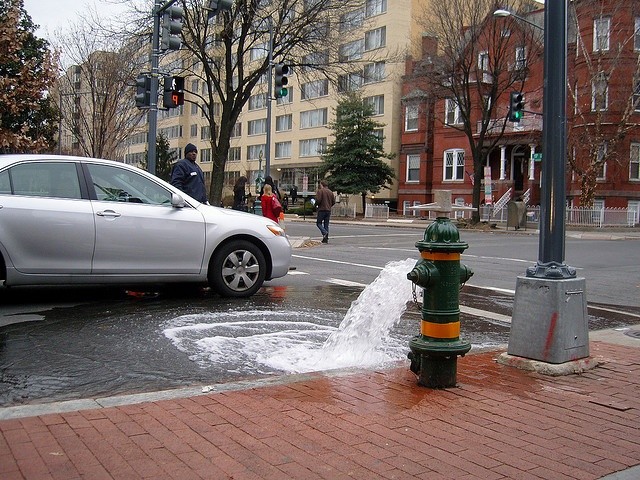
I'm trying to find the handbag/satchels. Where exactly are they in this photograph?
[271,196,283,218]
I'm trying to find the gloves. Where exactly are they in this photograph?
[280,212,284,220]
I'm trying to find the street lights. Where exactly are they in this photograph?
[494,10,544,30]
[57,90,110,155]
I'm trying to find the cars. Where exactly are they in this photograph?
[0,154,291,298]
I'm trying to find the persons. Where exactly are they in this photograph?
[282,194,289,214]
[260,176,282,203]
[261,185,283,223]
[313,181,336,243]
[290,184,297,207]
[171,144,208,205]
[245,192,255,212]
[232,176,247,211]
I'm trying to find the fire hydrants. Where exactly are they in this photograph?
[406,217,474,387]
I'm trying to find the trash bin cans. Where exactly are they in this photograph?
[254,200,262,216]
[507,202,527,230]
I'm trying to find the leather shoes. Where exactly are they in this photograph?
[321,232,329,243]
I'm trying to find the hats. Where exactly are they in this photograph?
[185,143,197,154]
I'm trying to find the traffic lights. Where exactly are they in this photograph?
[163,76,184,108]
[207,0,232,18]
[510,91,522,122]
[162,5,182,49]
[135,75,150,106]
[275,64,288,99]
